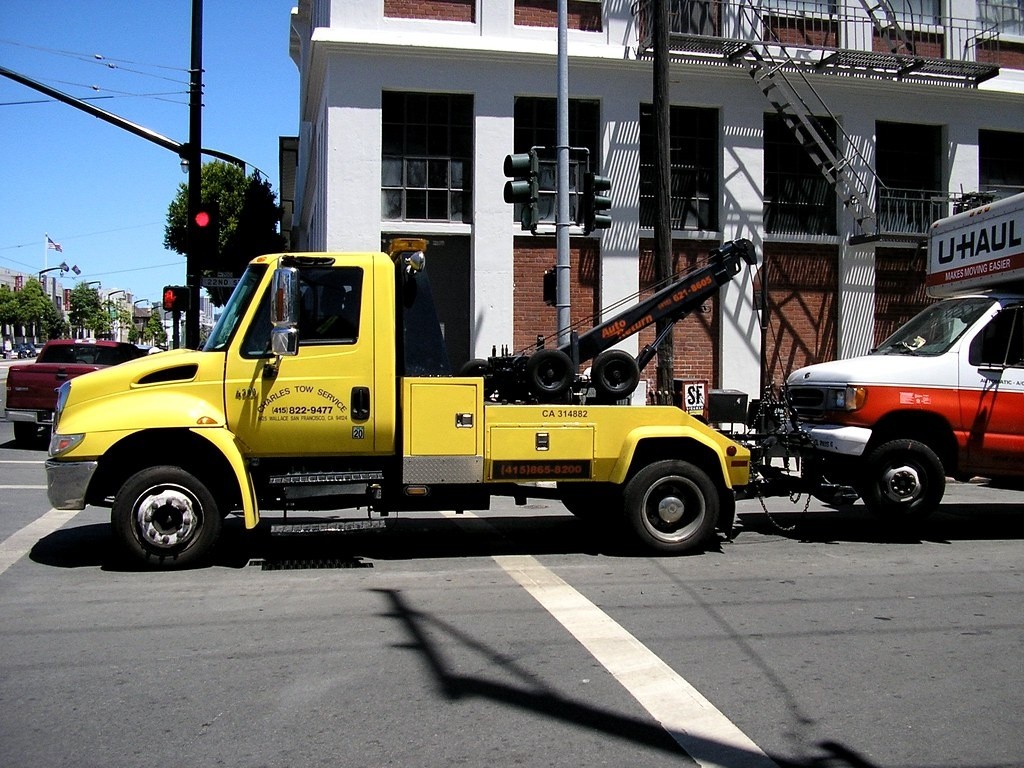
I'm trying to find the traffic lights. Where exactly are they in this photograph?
[583,170,632,237]
[161,284,189,313]
[189,205,221,249]
[502,151,539,233]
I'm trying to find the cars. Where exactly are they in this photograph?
[13,342,46,359]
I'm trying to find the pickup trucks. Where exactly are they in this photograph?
[5,339,144,442]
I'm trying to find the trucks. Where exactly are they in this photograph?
[784,190,1024,522]
[44,233,863,552]
